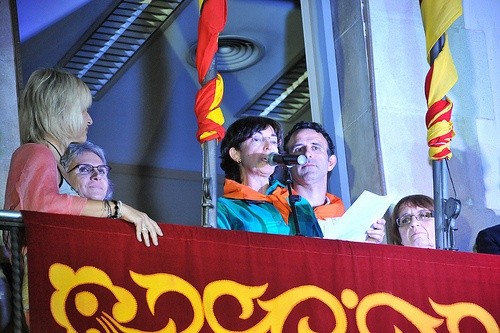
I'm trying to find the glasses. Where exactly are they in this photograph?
[395,209,433,226]
[67,163,111,176]
[248,134,283,146]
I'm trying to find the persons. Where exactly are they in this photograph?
[216,116,324,239]
[3,68,163,333]
[283,120,386,244]
[389,194,435,249]
[60,141,115,201]
[476,224,500,255]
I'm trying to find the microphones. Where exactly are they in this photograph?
[267,152,307,166]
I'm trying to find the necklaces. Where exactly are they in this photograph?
[42,138,62,162]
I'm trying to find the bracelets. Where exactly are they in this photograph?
[105,200,112,218]
[111,200,119,219]
[117,201,122,218]
